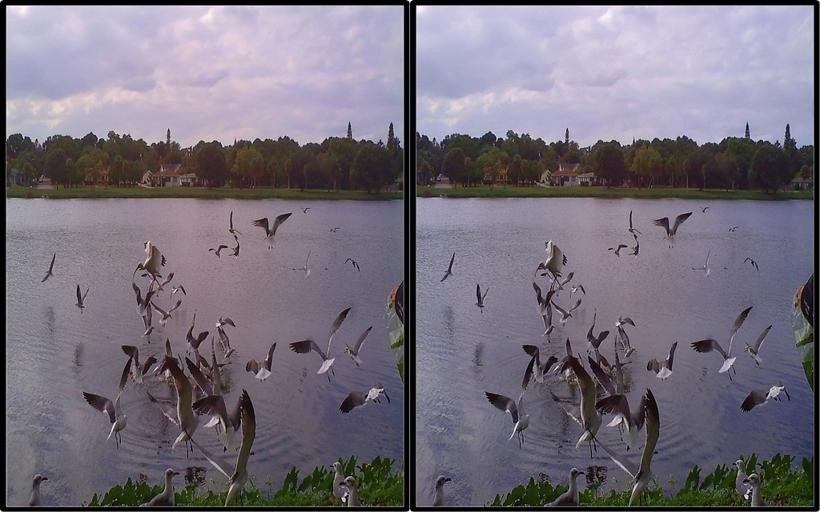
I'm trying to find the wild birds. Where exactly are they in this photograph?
[431,205,793,511]
[26,198,394,507]
